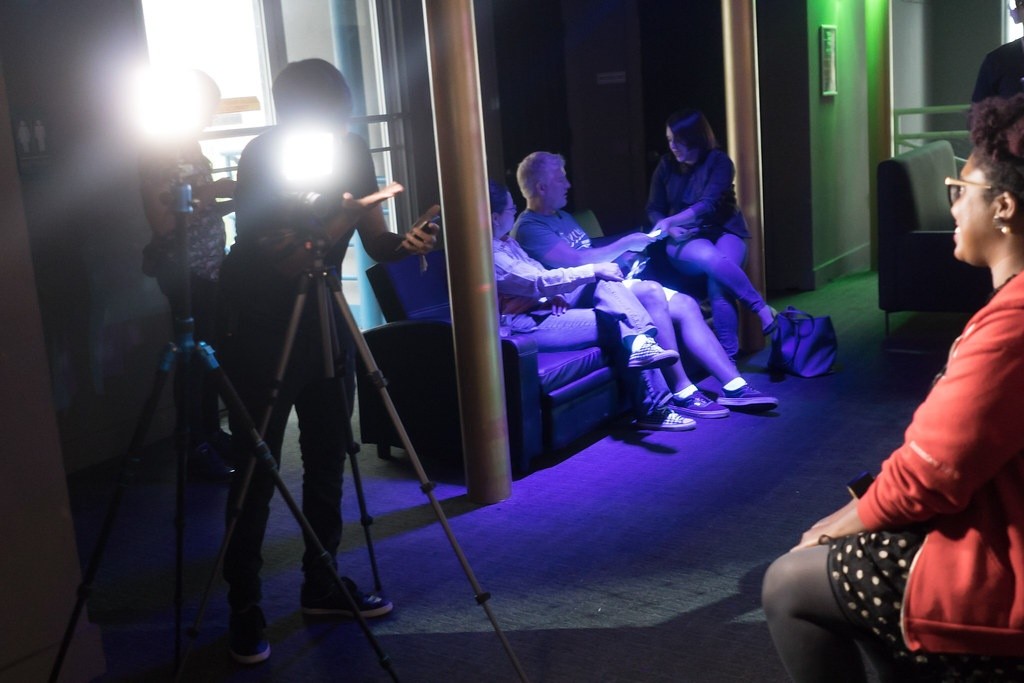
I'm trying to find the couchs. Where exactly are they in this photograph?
[875,141,994,336]
[355,208,744,478]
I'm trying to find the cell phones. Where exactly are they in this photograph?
[394,215,440,255]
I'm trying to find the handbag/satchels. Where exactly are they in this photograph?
[767,305,837,378]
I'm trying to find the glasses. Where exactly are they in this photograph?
[504,204,516,212]
[945,176,1018,206]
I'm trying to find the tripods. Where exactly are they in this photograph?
[48,173,531,683]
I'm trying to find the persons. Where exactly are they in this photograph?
[646,109,779,375]
[225,58,440,663]
[140,68,236,477]
[761,91,1024,683]
[490,151,779,432]
[972,0,1024,107]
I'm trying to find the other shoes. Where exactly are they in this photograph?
[179,441,236,480]
[204,427,238,463]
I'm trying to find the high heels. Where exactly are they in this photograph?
[758,303,777,335]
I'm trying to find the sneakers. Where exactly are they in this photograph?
[627,338,679,371]
[300,577,394,618]
[671,390,730,418]
[228,609,270,664]
[716,385,778,410]
[636,405,695,431]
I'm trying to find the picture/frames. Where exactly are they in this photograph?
[820,24,838,98]
